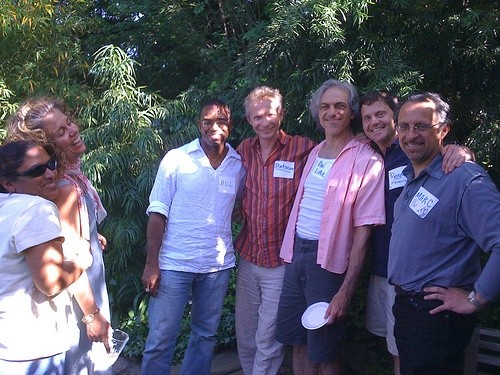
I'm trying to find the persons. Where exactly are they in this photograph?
[273,79,387,375]
[357,88,500,375]
[233,84,372,375]
[0,140,94,375]
[140,98,247,375]
[12,96,113,375]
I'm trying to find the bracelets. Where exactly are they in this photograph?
[82,308,99,324]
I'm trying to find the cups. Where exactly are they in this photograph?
[95,329,129,369]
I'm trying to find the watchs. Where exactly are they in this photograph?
[467,290,483,308]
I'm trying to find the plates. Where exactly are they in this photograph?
[301,302,330,330]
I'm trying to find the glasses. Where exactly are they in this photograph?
[395,122,441,132]
[17,157,59,178]
